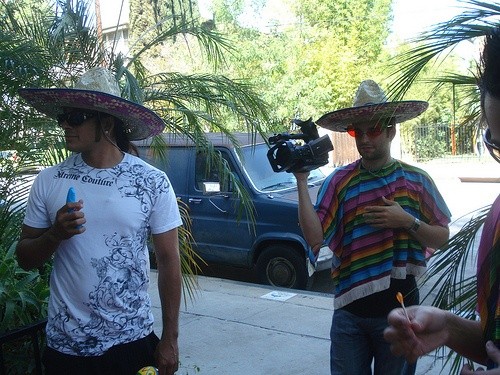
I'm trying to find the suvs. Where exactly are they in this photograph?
[121,133,338,289]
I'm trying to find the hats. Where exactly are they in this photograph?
[315,79,428,133]
[20,67,166,142]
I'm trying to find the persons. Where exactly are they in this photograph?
[293,79,454,375]
[385,23,500,375]
[15,67,182,375]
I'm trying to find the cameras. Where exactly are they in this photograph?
[267,116,333,173]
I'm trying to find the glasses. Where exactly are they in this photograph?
[55,109,96,129]
[481,128,500,162]
[345,123,393,139]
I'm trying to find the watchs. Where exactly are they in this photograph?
[408,218,420,234]
